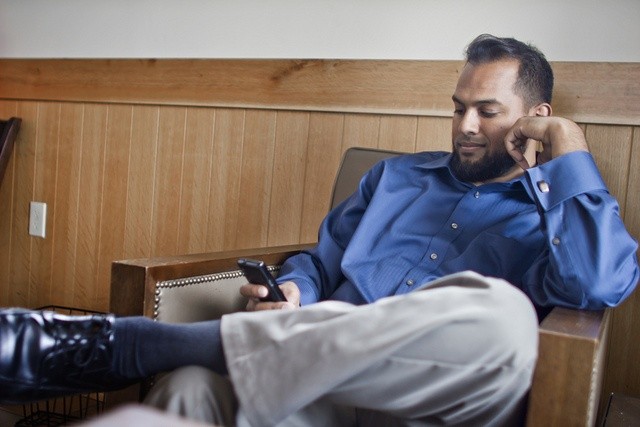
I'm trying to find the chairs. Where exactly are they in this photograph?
[110,147,611,426]
[1,115,22,178]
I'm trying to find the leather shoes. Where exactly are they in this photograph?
[1,306,135,401]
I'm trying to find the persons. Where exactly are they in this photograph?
[0,34,640,426]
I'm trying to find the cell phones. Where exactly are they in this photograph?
[238,258,287,303]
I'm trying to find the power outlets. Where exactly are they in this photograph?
[29,202,46,238]
